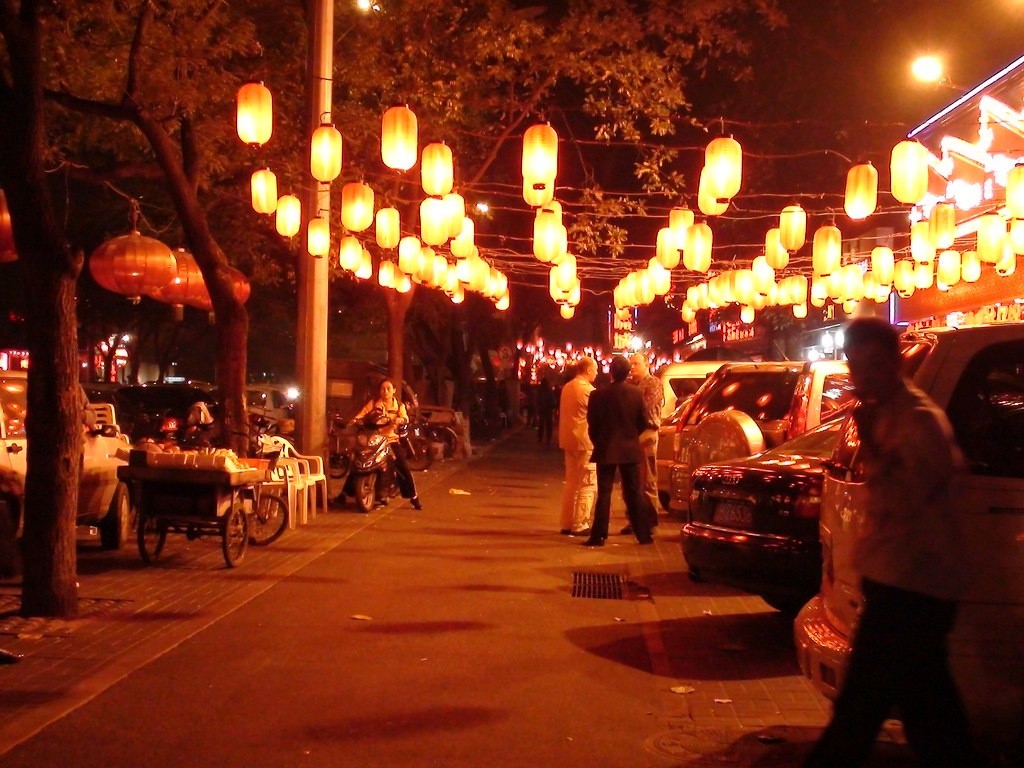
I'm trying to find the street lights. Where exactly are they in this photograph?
[908,53,971,96]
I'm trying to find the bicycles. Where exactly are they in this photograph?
[402,421,433,471]
[328,421,349,478]
[420,411,458,458]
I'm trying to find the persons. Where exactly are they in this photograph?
[581,354,657,547]
[80,380,96,467]
[558,355,600,536]
[792,316,984,768]
[496,375,565,444]
[328,378,423,512]
[619,353,664,536]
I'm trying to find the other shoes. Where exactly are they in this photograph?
[333,495,346,507]
[560,528,593,536]
[621,525,634,534]
[410,495,422,509]
[581,537,604,546]
[640,538,655,544]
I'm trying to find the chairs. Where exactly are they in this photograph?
[269,436,327,519]
[252,434,310,528]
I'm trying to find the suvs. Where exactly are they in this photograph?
[116,381,219,445]
[673,362,854,524]
[0,370,133,549]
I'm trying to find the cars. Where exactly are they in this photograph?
[680,415,846,613]
[655,400,695,510]
[246,387,293,436]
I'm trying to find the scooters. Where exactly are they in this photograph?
[345,414,413,513]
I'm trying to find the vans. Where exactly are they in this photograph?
[794,324,1023,749]
[657,362,777,427]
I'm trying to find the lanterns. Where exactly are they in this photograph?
[188,264,251,324]
[145,248,205,321]
[217,72,1024,387]
[86,228,178,304]
[0,188,19,266]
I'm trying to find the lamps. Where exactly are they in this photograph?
[232,70,1024,331]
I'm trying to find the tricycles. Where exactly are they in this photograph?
[116,423,289,569]
